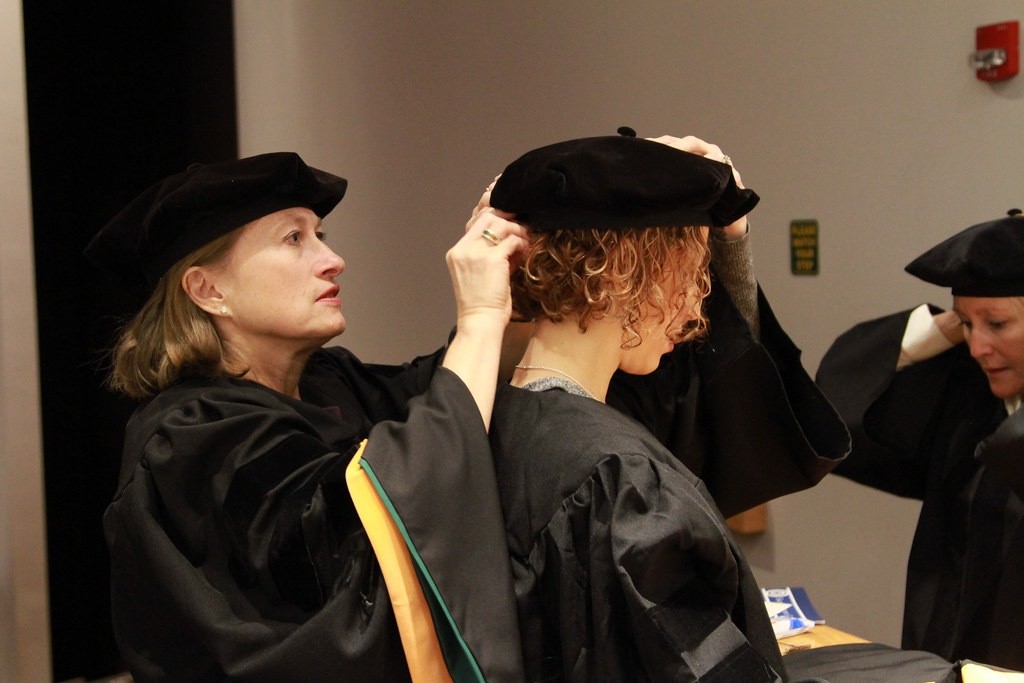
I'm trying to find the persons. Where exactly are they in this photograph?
[89,153,534,683]
[816,208,1024,674]
[491,126,851,682]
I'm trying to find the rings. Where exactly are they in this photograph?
[724,155,730,164]
[483,230,499,245]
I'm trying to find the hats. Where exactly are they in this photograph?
[489,125,761,235]
[903,208,1024,298]
[82,151,348,321]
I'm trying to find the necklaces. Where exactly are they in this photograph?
[515,366,596,399]
